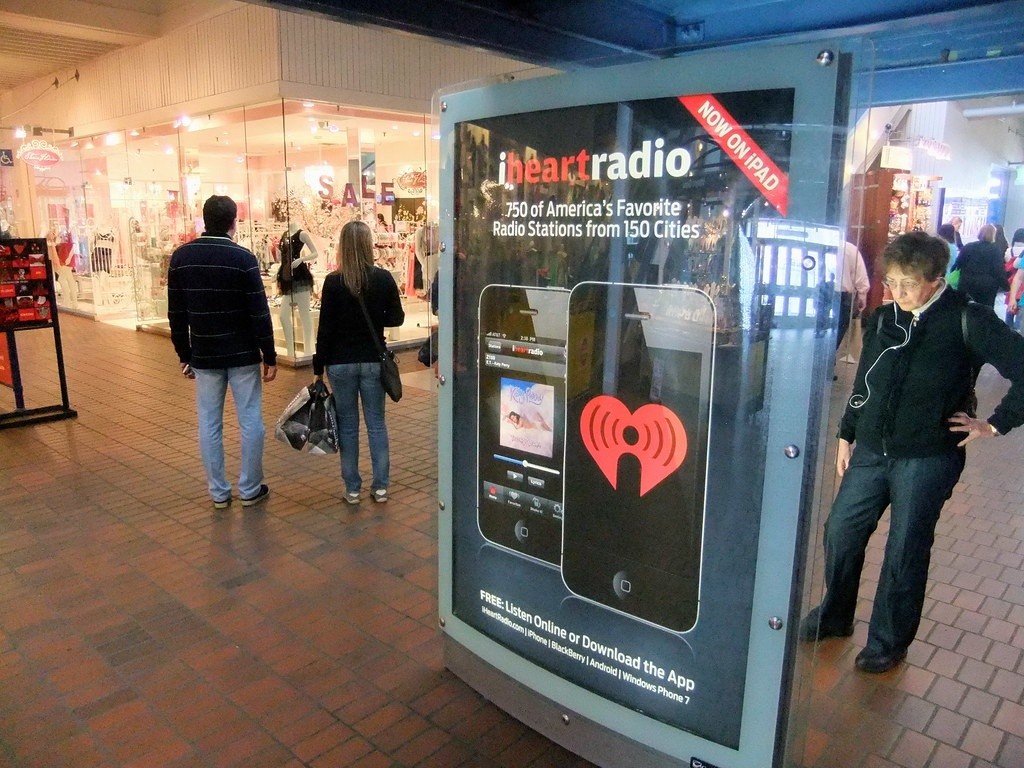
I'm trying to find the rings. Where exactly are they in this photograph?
[970,427,972,431]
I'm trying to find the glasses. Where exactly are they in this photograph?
[881,279,921,291]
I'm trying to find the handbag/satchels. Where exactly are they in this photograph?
[418,330,440,366]
[380,352,402,403]
[274,379,322,451]
[308,382,338,455]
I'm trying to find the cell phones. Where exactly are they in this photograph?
[477,281,717,635]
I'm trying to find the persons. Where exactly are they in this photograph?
[800,231,1024,672]
[279,220,318,356]
[833,242,870,381]
[378,213,388,232]
[0,219,16,239]
[414,221,426,264]
[166,195,278,509]
[419,259,439,367]
[37,220,78,309]
[503,411,551,432]
[312,221,404,503]
[939,216,1024,338]
[814,273,835,332]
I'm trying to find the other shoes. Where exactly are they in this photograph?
[800,611,854,643]
[342,488,360,504]
[374,489,388,502]
[855,645,909,673]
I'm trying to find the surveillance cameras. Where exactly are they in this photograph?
[886,123,892,130]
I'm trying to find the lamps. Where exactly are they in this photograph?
[305,147,334,186]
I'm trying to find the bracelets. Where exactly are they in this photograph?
[990,424,1000,436]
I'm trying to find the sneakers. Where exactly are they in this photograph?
[241,484,270,506]
[214,499,231,508]
